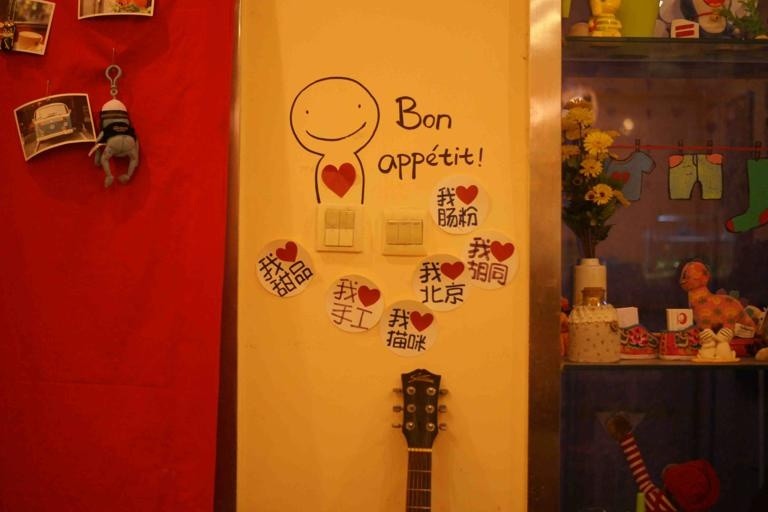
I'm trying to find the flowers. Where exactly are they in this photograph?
[561,93,631,258]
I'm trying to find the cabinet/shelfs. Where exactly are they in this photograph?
[561,0,768,512]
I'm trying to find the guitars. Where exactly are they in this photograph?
[392,367,449,512]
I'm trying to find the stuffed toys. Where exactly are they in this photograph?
[95,96,142,188]
[596,409,722,512]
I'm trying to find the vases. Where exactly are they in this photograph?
[573,258,608,306]
